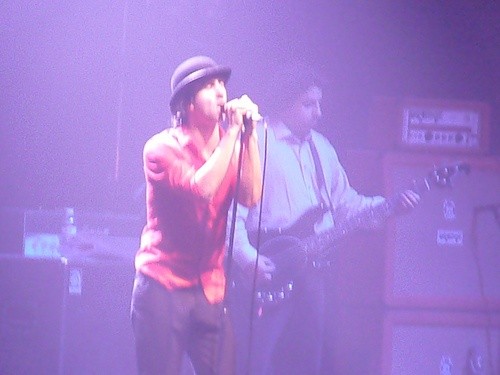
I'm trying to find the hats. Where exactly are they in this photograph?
[169,56,231,112]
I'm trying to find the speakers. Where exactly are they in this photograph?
[337,151,500,312]
[325,309,500,375]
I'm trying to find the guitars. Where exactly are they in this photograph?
[227,163,456,308]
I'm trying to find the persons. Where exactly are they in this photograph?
[128,56,264,374]
[222,62,422,375]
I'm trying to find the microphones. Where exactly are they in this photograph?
[223,103,263,122]
